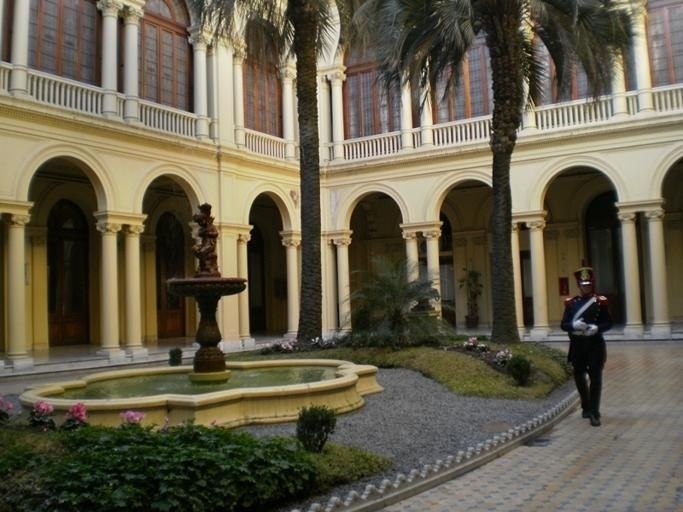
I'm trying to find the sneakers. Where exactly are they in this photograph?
[583,409,600,426]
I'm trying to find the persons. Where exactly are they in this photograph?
[559,267,614,427]
[191,202,221,277]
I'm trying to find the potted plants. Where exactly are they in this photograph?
[457,267,484,329]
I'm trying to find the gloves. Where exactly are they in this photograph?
[573,318,587,330]
[588,323,598,336]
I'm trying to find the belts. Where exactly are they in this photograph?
[571,330,584,336]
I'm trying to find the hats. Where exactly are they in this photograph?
[574,268,595,288]
